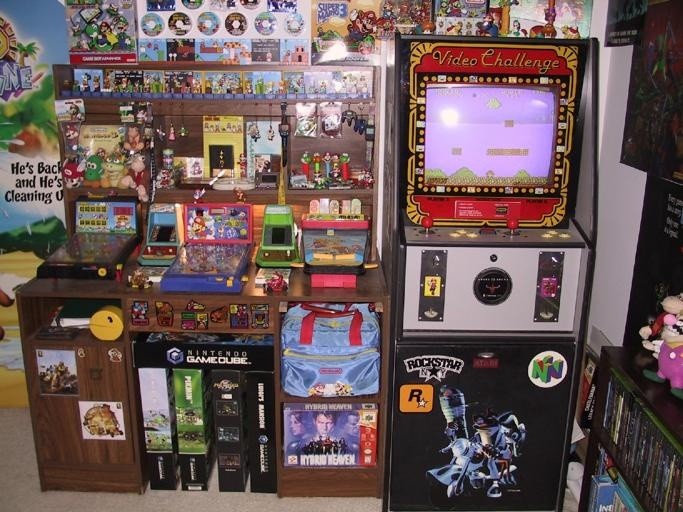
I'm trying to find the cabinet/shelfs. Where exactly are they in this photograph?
[574,343,682,511]
[49,58,383,263]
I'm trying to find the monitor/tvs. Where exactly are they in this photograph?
[400,40,589,229]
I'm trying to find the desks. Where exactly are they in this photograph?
[11,238,391,497]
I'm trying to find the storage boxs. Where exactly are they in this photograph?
[132,333,277,496]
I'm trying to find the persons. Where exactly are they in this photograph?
[286,410,361,456]
